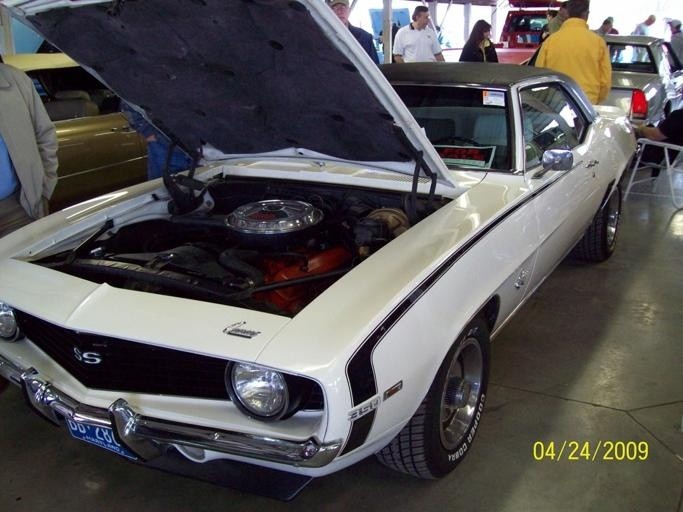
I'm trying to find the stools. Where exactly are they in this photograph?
[622,136,682,212]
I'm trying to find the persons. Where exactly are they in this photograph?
[459,20,498,63]
[0,63,59,237]
[119,101,188,180]
[535,0,683,169]
[393,6,445,63]
[327,0,379,64]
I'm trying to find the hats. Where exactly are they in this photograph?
[329,0,349,7]
[667,20,681,28]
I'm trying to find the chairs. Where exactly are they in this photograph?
[463,113,540,174]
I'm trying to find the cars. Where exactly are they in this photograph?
[1,0,640,507]
[1,51,155,218]
[596,35,683,141]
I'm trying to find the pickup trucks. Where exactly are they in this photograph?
[484,6,572,68]
